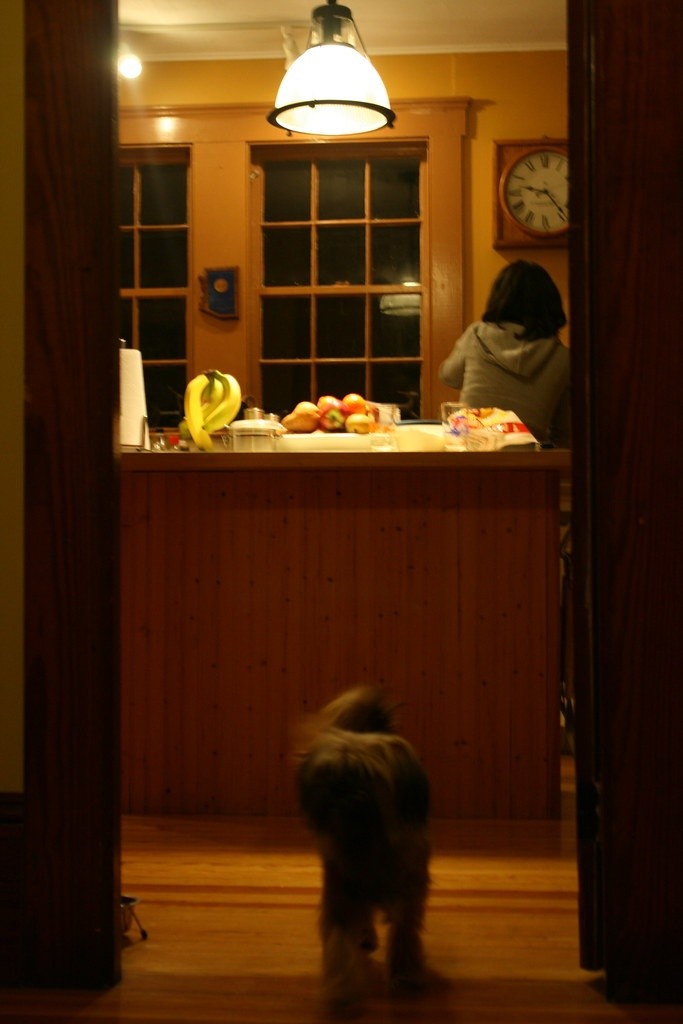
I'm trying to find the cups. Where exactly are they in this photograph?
[243,407,264,420]
[265,413,280,422]
[440,401,469,451]
[365,403,397,452]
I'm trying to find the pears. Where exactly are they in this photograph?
[281,402,320,433]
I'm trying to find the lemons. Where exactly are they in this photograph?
[317,393,368,414]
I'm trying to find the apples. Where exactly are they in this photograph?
[319,400,379,434]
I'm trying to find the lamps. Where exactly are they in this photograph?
[266,0,397,135]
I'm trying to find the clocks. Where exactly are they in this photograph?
[489,137,570,249]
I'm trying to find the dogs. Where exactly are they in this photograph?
[291,684,436,997]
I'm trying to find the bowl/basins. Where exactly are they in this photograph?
[227,419,287,453]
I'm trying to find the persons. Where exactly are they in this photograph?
[437,258,576,450]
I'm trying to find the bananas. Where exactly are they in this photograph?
[180,369,242,452]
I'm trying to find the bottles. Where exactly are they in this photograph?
[151,428,170,452]
